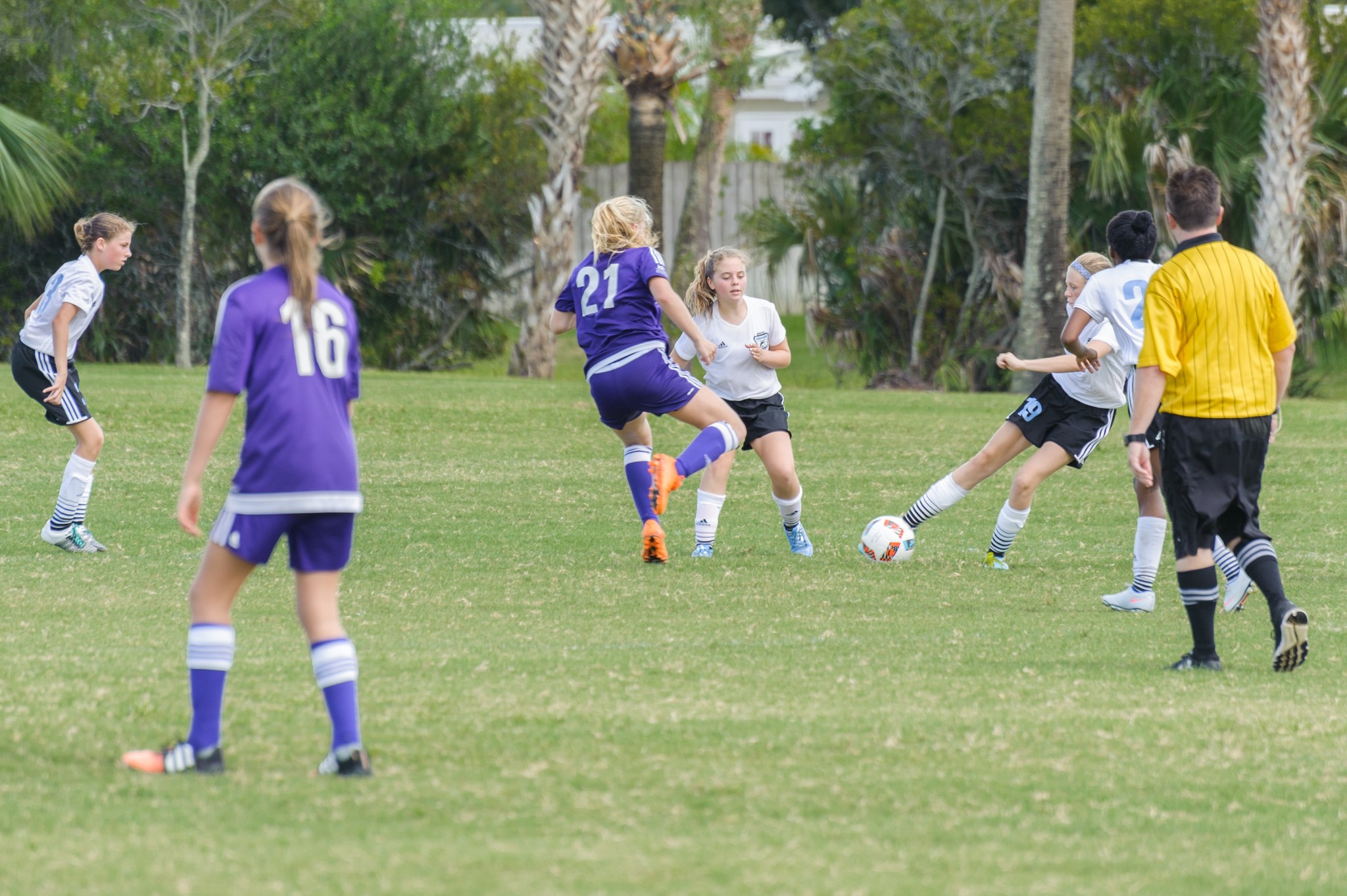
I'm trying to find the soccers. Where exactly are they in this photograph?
[861,515,915,564]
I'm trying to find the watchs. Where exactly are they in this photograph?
[1124,433,1146,446]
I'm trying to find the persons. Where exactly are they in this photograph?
[11,212,135,553]
[857,251,1129,570]
[1060,211,1252,611]
[1128,166,1308,673]
[549,196,746,564]
[121,178,374,778]
[668,246,814,559]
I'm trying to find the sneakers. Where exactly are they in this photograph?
[648,453,685,515]
[307,749,372,778]
[1170,652,1221,673]
[40,519,98,554]
[75,524,107,551]
[782,519,813,557]
[981,552,1008,571]
[1269,603,1309,672]
[1223,565,1257,613]
[1101,582,1155,613]
[691,544,713,558]
[641,519,669,564]
[121,732,224,775]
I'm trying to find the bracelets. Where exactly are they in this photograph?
[1273,409,1277,414]
